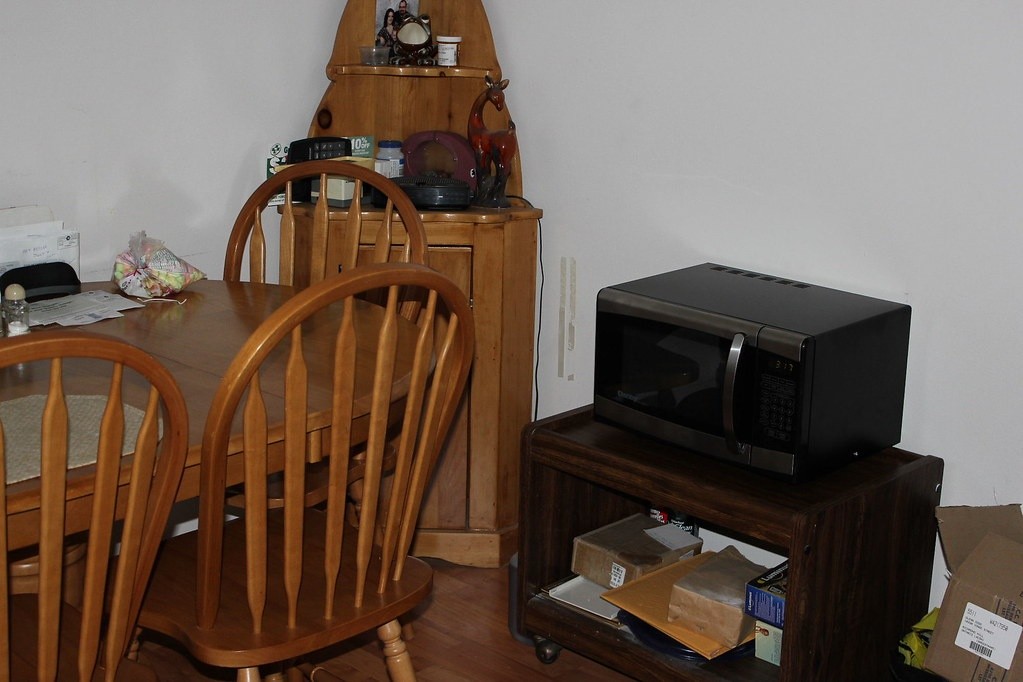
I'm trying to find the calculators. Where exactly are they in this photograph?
[287,136,352,203]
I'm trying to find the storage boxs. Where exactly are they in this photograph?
[570,513,703,590]
[744,559,790,629]
[922,503,1023,682]
[668,545,769,649]
[755,619,783,667]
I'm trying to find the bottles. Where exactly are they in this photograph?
[374,141,405,179]
[436,35,462,68]
[1,284,31,373]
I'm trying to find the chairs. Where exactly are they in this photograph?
[98,262,474,682]
[0,333,189,682]
[225,161,428,640]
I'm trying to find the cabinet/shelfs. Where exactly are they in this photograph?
[516,403,944,682]
[277,0,544,568]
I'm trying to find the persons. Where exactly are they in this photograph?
[376,0,412,48]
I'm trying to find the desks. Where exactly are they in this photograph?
[0,280,436,605]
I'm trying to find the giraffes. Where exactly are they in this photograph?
[467,74,517,209]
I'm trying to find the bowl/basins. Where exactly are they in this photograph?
[358,46,391,66]
